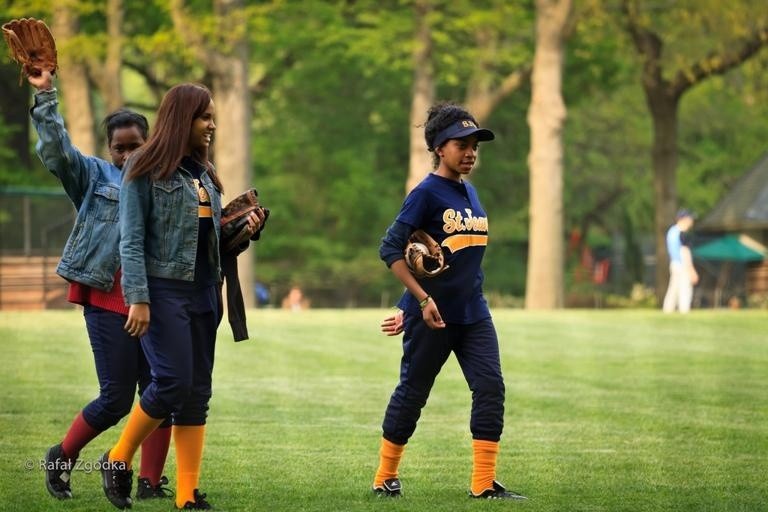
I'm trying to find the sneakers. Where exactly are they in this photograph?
[97,449,134,510]
[135,475,175,499]
[369,479,404,498]
[173,488,221,510]
[468,480,527,499]
[44,441,78,500]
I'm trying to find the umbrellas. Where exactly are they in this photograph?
[685,232,767,263]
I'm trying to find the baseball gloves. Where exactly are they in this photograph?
[219,186,272,250]
[402,229,450,276]
[0,15,59,76]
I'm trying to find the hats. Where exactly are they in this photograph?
[431,118,495,148]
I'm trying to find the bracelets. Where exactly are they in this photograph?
[419,294,433,311]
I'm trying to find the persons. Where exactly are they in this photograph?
[99,81,269,509]
[372,100,528,501]
[26,69,173,501]
[662,207,700,311]
[280,285,312,309]
